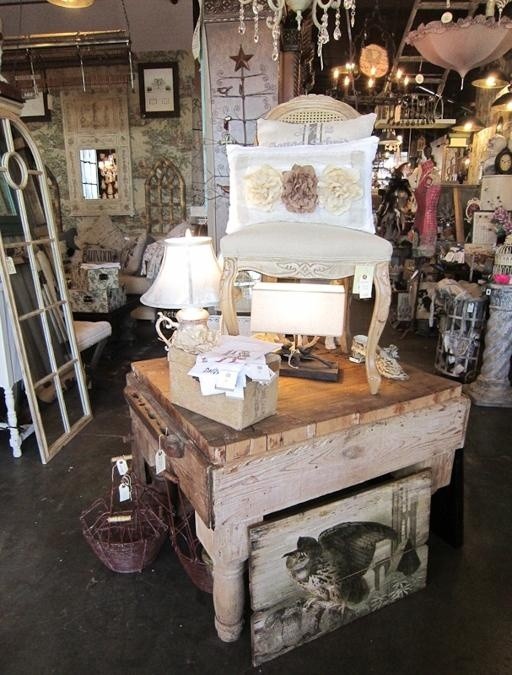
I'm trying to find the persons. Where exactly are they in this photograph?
[98,150,119,200]
[412,157,440,238]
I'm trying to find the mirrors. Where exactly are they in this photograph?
[80,148,118,200]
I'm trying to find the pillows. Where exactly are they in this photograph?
[255,112,379,145]
[227,142,379,236]
[76,210,137,256]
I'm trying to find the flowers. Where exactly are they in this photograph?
[492,200,511,234]
[481,263,512,292]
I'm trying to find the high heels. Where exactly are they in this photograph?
[350,334,410,382]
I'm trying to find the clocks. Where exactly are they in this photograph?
[493,148,512,175]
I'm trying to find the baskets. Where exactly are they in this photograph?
[81,465,168,575]
[435,289,491,383]
[162,473,215,593]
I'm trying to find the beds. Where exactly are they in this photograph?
[63,218,205,298]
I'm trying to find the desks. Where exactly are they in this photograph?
[123,336,473,640]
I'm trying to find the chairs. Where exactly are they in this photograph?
[219,89,395,394]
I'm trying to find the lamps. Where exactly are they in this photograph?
[99,154,117,197]
[140,226,221,363]
[470,60,507,90]
[238,1,359,71]
[490,91,512,117]
[334,33,412,95]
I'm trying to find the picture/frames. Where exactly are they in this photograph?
[140,63,180,119]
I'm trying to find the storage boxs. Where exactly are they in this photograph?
[66,280,127,313]
[169,327,282,430]
[72,264,119,292]
